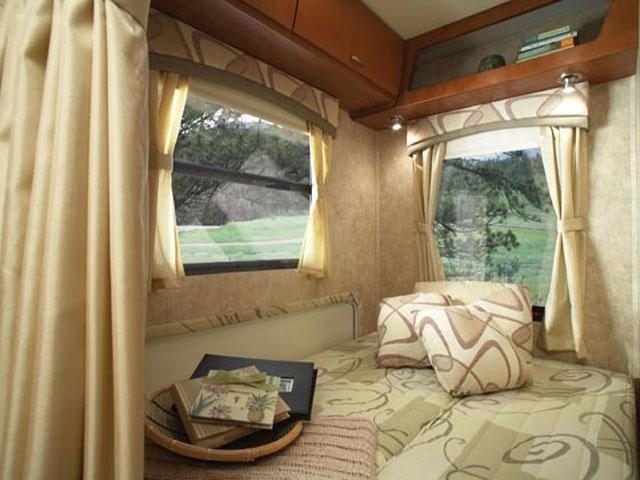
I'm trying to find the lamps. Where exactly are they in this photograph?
[561,76,578,95]
[389,116,405,131]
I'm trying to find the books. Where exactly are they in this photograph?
[514,24,578,64]
[170,353,318,447]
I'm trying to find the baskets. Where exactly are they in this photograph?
[143,377,303,462]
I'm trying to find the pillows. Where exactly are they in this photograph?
[407,303,533,396]
[415,282,533,368]
[377,293,452,368]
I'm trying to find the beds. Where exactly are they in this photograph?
[144,290,636,480]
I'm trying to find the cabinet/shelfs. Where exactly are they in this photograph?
[151,2,405,115]
[400,0,638,120]
